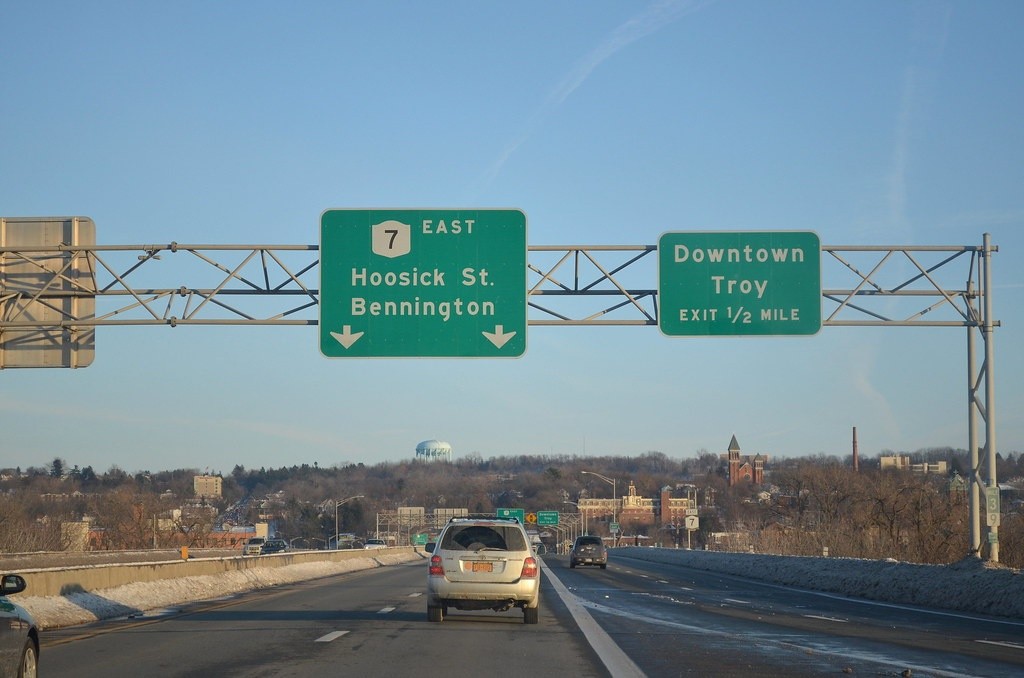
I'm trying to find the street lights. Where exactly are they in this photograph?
[335,495,365,550]
[564,501,588,537]
[581,471,616,547]
[312,538,327,549]
[291,537,303,550]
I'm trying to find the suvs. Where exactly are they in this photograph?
[425,515,547,624]
[569,536,608,568]
[243,537,268,555]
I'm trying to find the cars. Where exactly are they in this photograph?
[0,573,41,678]
[330,540,363,549]
[363,538,388,550]
[259,540,291,555]
